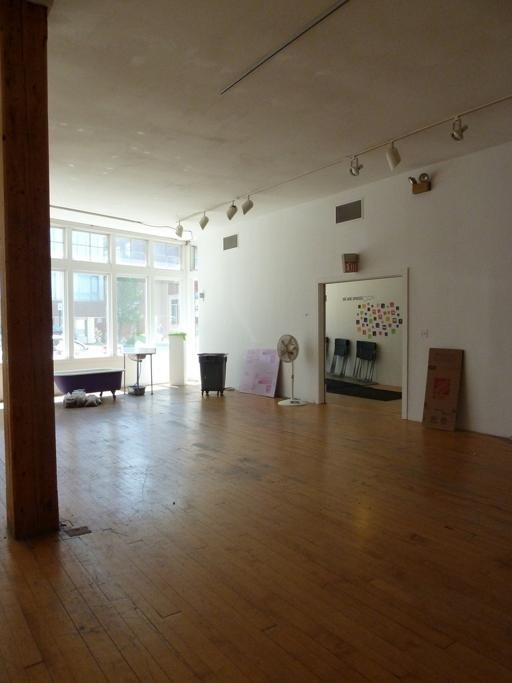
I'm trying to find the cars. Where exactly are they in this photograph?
[53,334,90,358]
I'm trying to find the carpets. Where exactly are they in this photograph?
[324,378,402,402]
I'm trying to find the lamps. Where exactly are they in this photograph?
[173,190,254,237]
[347,115,468,195]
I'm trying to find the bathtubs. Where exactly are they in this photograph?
[53,368,125,394]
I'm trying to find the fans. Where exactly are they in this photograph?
[277,334,308,406]
